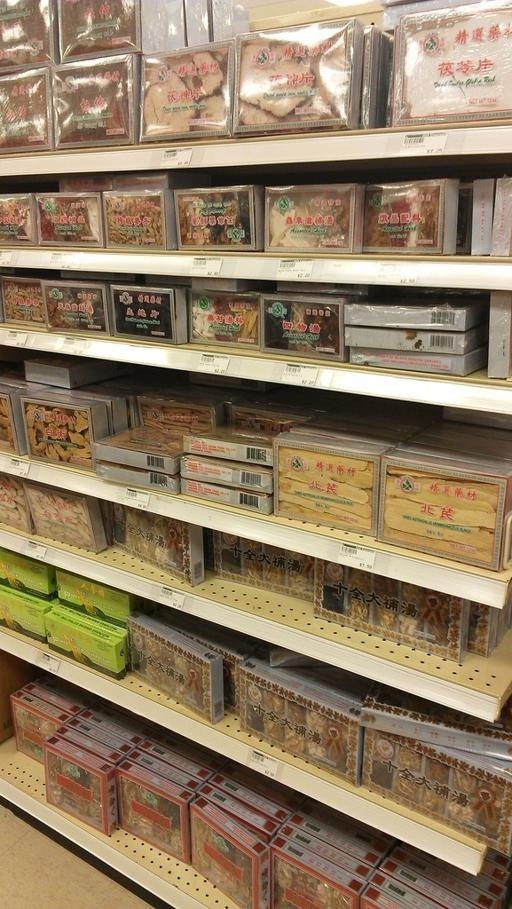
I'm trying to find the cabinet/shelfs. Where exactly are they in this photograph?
[1,0,509,908]
